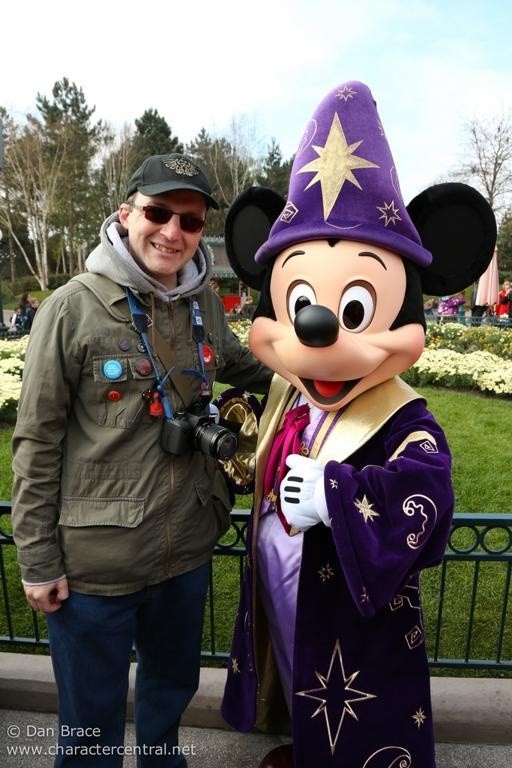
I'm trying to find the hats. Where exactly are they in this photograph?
[125,153,220,210]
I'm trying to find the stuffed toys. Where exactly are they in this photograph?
[217,78,500,768]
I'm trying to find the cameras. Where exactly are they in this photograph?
[161,387,240,462]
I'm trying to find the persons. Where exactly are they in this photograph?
[10,306,25,328]
[19,291,33,316]
[501,284,512,319]
[3,151,274,768]
[496,280,511,314]
[435,291,466,327]
[470,280,487,326]
[27,297,42,330]
[240,286,253,307]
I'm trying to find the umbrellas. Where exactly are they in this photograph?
[473,242,500,318]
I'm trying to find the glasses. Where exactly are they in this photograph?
[129,202,205,233]
